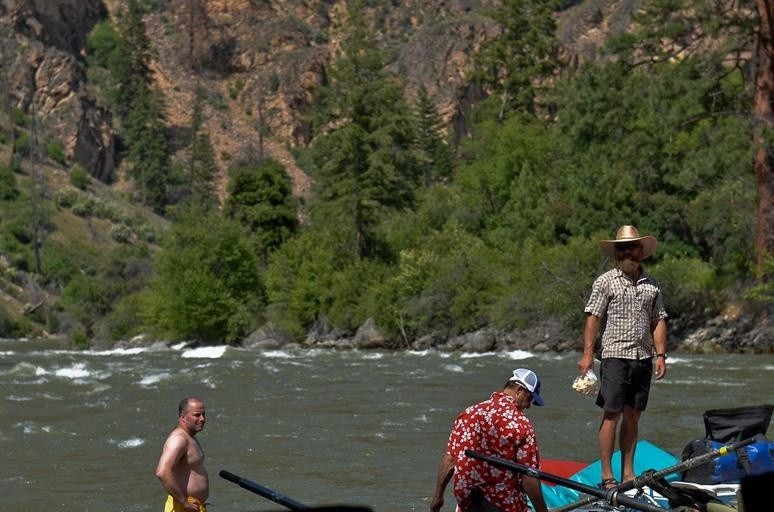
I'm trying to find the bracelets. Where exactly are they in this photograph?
[656,351,668,359]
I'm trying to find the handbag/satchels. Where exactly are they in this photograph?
[679,434,773,485]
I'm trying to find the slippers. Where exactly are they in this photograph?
[601,478,619,491]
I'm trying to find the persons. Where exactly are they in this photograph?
[151,396,217,511]
[425,367,551,511]
[575,222,669,494]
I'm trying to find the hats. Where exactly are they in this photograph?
[599,224,658,259]
[507,367,545,407]
